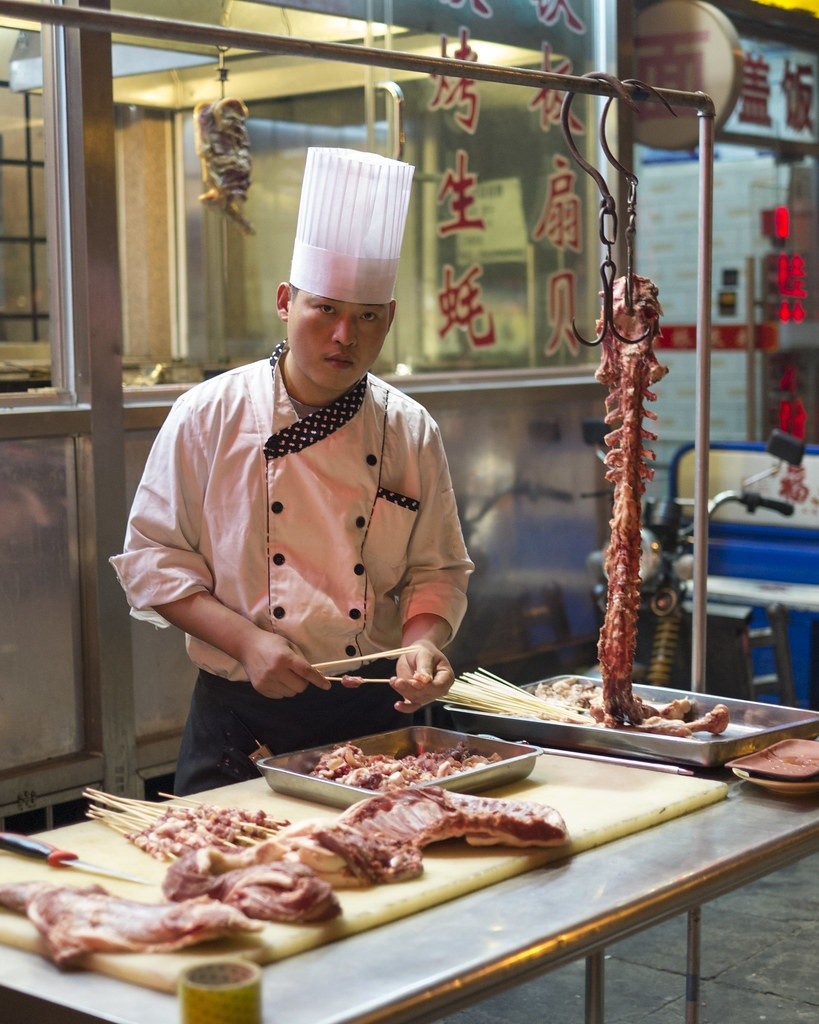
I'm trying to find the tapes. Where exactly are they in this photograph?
[179,957,269,1023]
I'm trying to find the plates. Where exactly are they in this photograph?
[731,768,819,795]
[724,739,819,779]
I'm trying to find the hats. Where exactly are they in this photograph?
[289,146,416,305]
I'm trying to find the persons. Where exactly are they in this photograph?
[107,147,475,799]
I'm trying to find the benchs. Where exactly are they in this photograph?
[684,571,819,707]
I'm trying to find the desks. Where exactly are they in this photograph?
[1,731,819,1024]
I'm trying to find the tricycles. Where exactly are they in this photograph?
[580,418,818,713]
[414,406,613,698]
